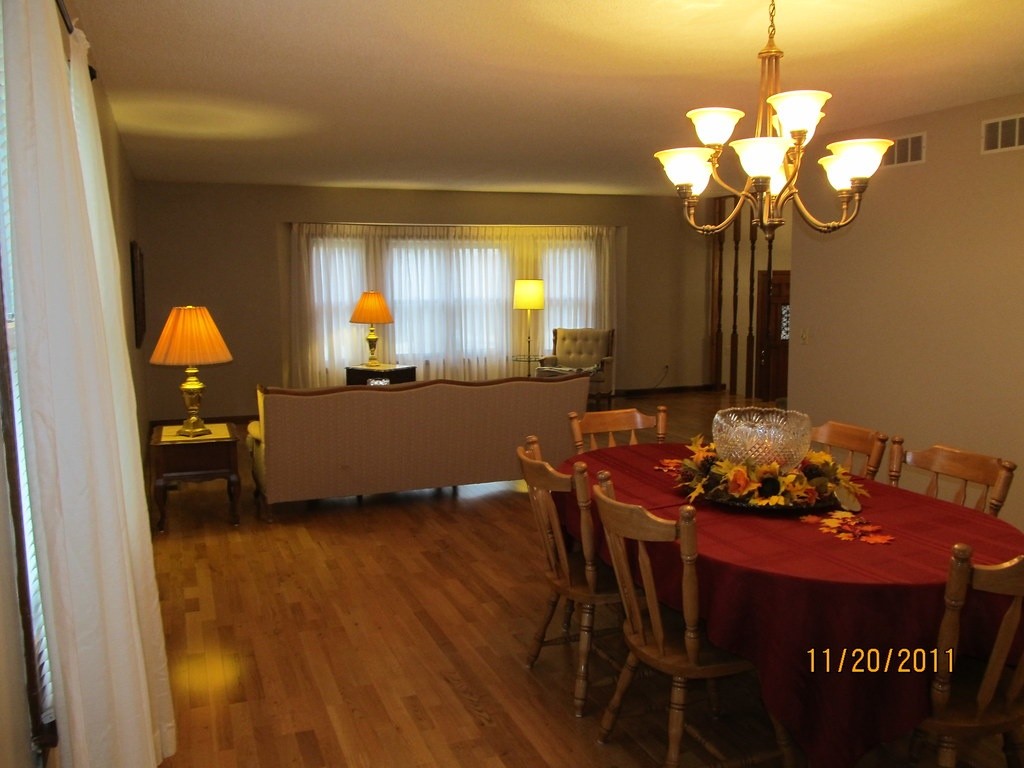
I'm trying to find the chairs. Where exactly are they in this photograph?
[908,541,1023,768]
[885,437,1017,519]
[592,469,800,768]
[565,405,668,455]
[811,421,889,485]
[513,434,647,720]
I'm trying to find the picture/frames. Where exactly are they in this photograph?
[131,240,148,350]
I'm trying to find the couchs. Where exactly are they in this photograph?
[244,371,597,515]
[537,328,615,412]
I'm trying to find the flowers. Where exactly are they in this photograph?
[654,433,871,511]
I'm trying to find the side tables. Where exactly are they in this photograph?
[147,422,241,534]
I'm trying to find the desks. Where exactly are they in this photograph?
[344,363,417,386]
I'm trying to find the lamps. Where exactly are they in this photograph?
[512,279,545,376]
[349,291,394,367]
[147,287,235,439]
[653,0,894,242]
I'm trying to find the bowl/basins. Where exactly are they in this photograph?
[715,408,810,476]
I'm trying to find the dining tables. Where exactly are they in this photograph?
[554,440,1024,768]
